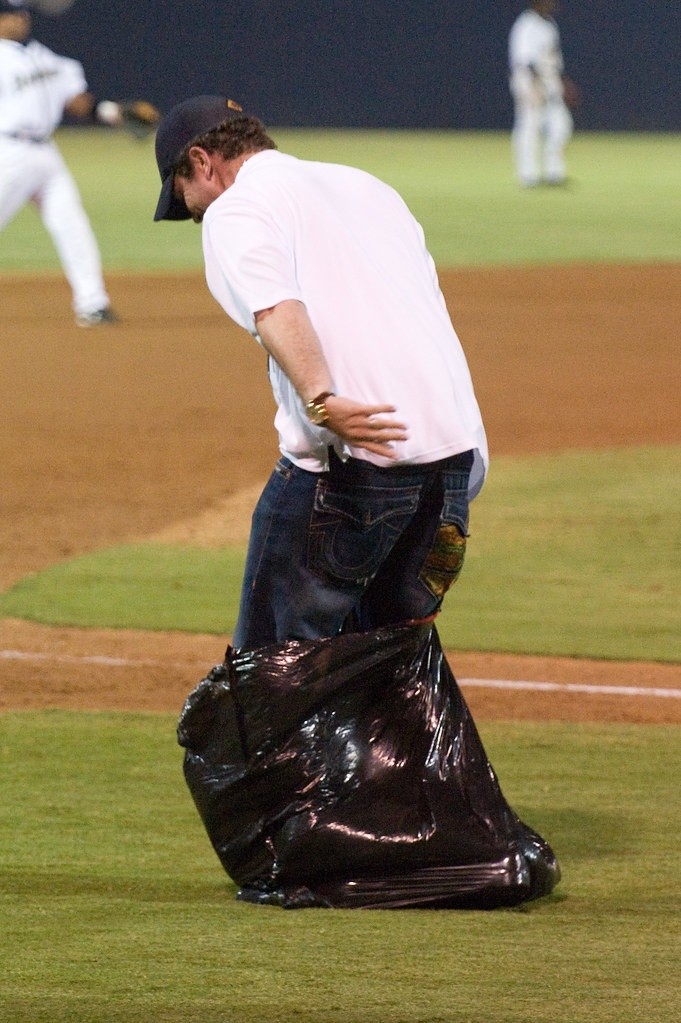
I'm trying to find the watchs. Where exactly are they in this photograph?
[303,392,337,426]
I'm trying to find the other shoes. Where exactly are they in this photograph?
[77,309,112,327]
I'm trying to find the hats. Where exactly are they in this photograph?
[154,95,248,222]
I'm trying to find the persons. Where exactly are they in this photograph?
[0,0,159,324]
[505,0,579,188]
[152,91,490,661]
